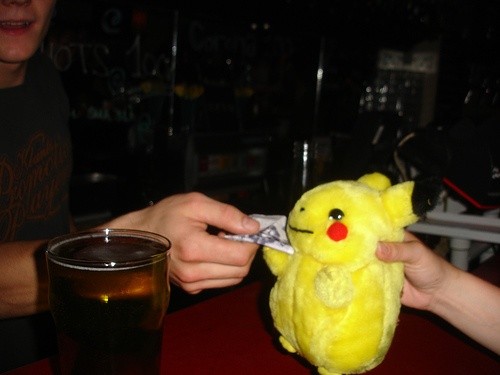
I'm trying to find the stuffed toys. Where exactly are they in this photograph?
[262,170,421,375]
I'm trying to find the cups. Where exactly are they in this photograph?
[46,228,173,375]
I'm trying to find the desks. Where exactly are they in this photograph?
[0,280,499,375]
[404,204,500,274]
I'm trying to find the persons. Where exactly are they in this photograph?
[0,0,259,371]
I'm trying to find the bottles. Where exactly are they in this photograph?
[288,141,318,212]
